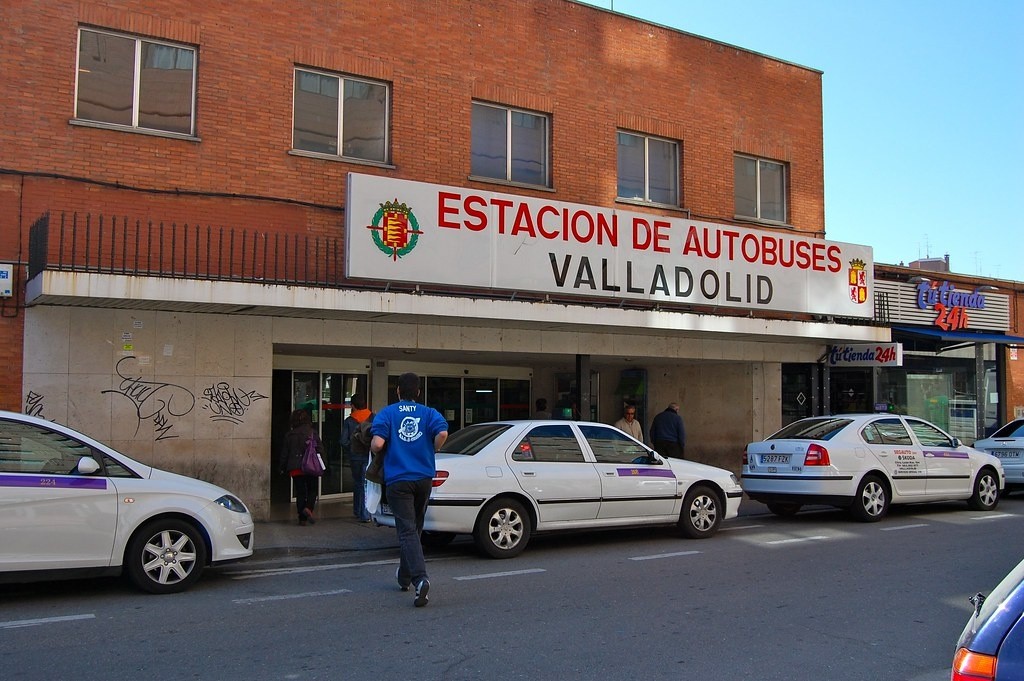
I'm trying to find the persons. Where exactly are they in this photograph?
[370,372,448,607]
[650,402,685,460]
[532,398,552,420]
[280,409,328,525]
[614,404,643,443]
[340,394,376,521]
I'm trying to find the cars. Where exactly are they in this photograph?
[968,418,1024,497]
[741,412,1006,524]
[0,410,254,595]
[371,419,744,560]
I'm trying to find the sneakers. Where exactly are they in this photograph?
[413,580,430,607]
[400,582,411,591]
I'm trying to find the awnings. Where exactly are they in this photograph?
[892,326,1024,344]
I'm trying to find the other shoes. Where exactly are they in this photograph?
[300,520,306,526]
[303,507,315,523]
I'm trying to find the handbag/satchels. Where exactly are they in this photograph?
[365,449,382,514]
[301,429,325,476]
